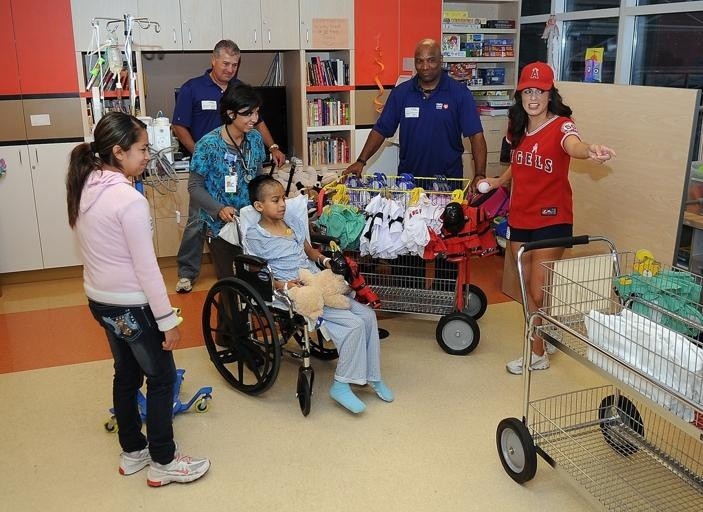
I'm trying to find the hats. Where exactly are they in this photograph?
[517,62,554,91]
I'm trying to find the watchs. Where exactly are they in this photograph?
[268,143,279,151]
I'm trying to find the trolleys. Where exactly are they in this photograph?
[310,176,486,355]
[497,235,703,511]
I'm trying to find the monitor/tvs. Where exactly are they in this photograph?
[175,85,289,154]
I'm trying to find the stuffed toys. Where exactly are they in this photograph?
[284,267,351,320]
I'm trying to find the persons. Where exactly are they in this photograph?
[66,113,212,487]
[342,37,488,196]
[171,40,286,294]
[476,62,616,375]
[187,83,266,281]
[242,174,394,414]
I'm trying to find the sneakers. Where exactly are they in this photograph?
[176,278,194,293]
[119,440,179,475]
[148,456,211,487]
[506,351,550,375]
[544,341,555,354]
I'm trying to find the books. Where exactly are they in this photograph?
[306,95,350,127]
[304,55,350,86]
[86,56,136,91]
[307,136,350,166]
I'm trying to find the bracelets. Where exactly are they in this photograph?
[355,157,367,166]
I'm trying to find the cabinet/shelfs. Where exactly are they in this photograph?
[0,142,84,274]
[138,0,222,52]
[75,51,146,142]
[282,51,355,166]
[70,0,141,52]
[135,170,354,258]
[1,96,84,142]
[1,0,80,96]
[355,88,392,128]
[222,0,300,51]
[355,128,400,176]
[355,0,442,88]
[299,0,355,50]
[442,0,522,100]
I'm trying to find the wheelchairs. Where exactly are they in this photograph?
[202,215,350,396]
